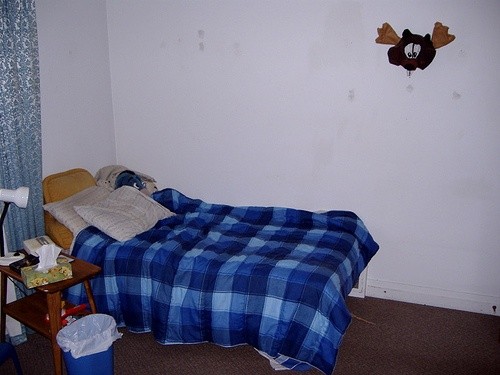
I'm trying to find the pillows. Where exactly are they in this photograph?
[41,183,114,237]
[73,185,175,242]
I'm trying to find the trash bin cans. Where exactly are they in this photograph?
[56,314,123,375]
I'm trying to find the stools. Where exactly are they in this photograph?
[0,246,102,374]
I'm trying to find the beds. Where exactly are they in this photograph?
[41,165,379,373]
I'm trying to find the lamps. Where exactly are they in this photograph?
[0,185,30,266]
